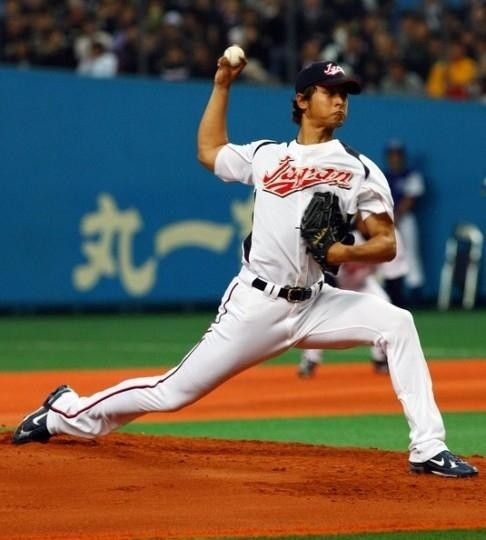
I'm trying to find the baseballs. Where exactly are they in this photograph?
[222,46,244,67]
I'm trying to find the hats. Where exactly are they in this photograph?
[295,61,362,96]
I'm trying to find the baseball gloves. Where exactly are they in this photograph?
[301,190,354,275]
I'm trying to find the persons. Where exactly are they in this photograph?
[1,1,486,99]
[297,344,389,379]
[14,46,481,479]
[381,142,427,312]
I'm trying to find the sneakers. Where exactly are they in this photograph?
[409,448,480,478]
[11,385,72,445]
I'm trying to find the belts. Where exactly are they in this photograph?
[252,277,323,302]
